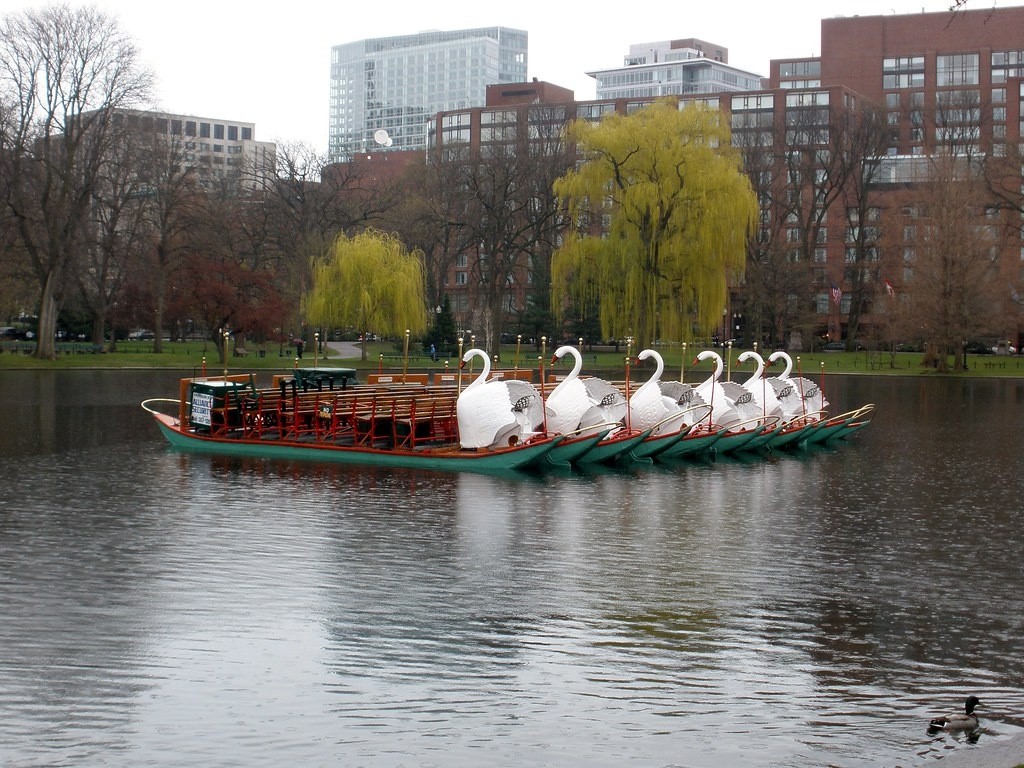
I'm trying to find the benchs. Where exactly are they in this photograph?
[214,381,643,452]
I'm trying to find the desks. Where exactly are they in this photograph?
[294,368,356,433]
[192,381,245,435]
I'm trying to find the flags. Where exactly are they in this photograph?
[831,281,841,305]
[885,274,896,297]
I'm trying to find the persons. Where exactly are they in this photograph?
[431,344,435,361]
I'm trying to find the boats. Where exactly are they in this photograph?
[142,345,877,475]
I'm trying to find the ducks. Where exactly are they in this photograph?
[928,696,984,733]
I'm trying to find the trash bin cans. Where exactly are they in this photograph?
[258,349,265,358]
